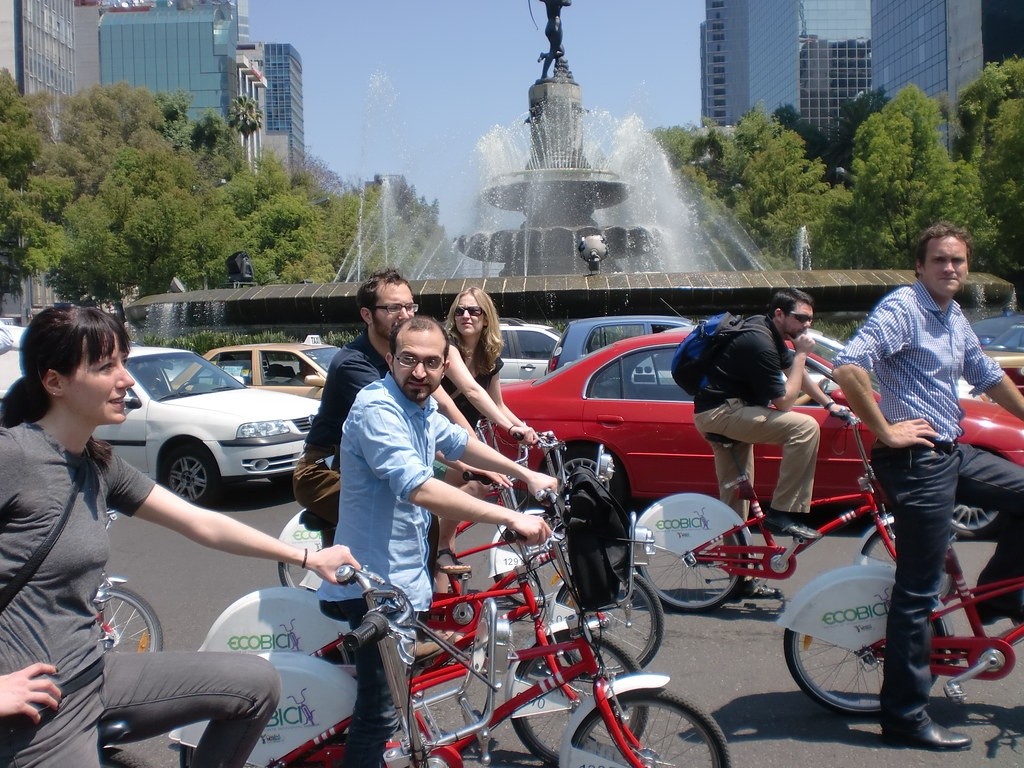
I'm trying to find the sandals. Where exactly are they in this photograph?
[435,549,472,574]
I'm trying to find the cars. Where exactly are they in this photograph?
[171,334,343,401]
[88,346,321,511]
[480,330,1023,540]
[496,306,1023,392]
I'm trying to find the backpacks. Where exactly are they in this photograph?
[671,311,777,397]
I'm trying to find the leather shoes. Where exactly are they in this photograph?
[975,598,1024,626]
[748,583,783,600]
[763,513,823,539]
[881,718,972,752]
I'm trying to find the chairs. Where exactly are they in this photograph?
[267,364,285,381]
[283,366,296,379]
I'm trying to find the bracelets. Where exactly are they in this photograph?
[824,400,834,410]
[508,425,515,433]
[303,549,307,565]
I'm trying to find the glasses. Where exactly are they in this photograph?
[454,306,486,316]
[367,303,420,314]
[788,311,816,323]
[391,353,448,372]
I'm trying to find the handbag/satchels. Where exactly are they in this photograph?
[560,465,632,611]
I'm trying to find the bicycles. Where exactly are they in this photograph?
[98,431,739,767]
[782,533,1024,717]
[91,506,164,654]
[632,406,955,615]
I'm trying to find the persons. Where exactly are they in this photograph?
[537,0,572,79]
[435,287,538,595]
[0,305,361,768]
[294,358,315,383]
[294,270,512,587]
[795,379,829,405]
[834,224,1024,751]
[693,288,850,597]
[318,318,557,767]
